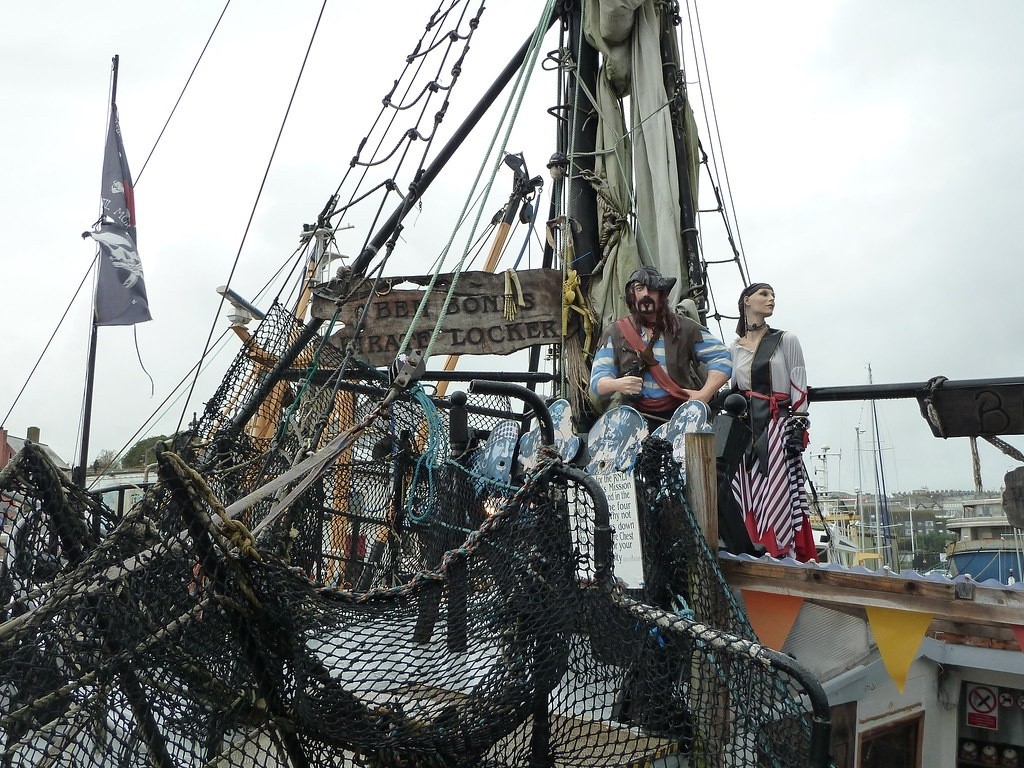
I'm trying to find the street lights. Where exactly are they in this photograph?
[894,502,915,562]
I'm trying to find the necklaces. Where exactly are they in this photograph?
[746,321,766,332]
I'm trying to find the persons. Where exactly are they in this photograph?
[590,266,731,438]
[728,283,821,563]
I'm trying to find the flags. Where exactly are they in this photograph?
[81,98,151,324]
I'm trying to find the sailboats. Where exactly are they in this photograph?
[807,446,860,566]
[944,437,1024,586]
